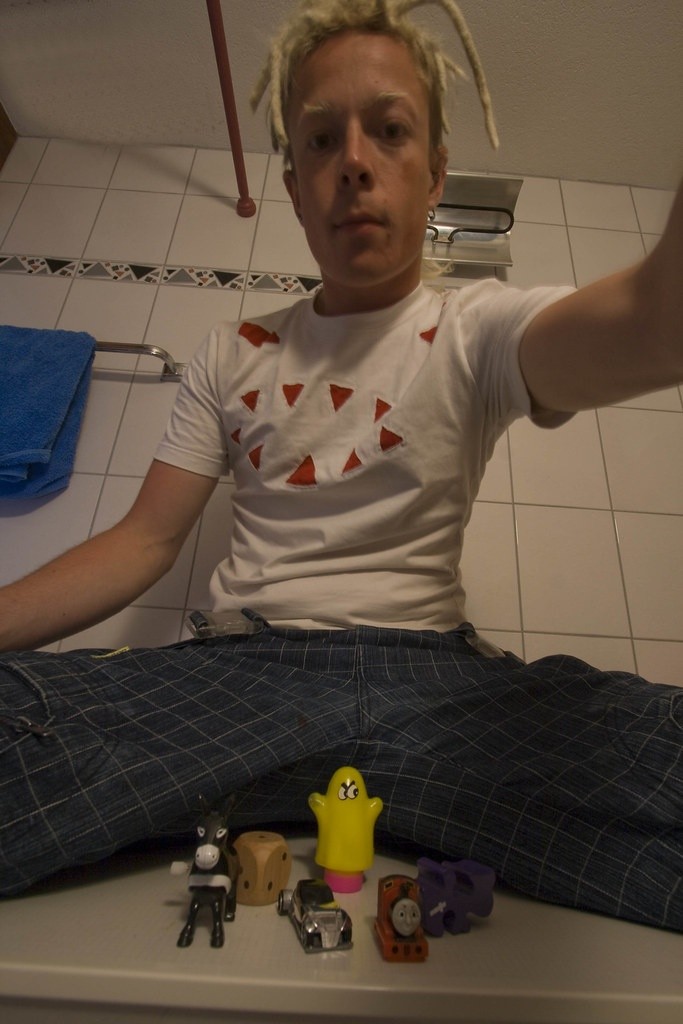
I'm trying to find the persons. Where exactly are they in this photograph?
[0,0,683,937]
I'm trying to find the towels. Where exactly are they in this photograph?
[0,322,97,502]
